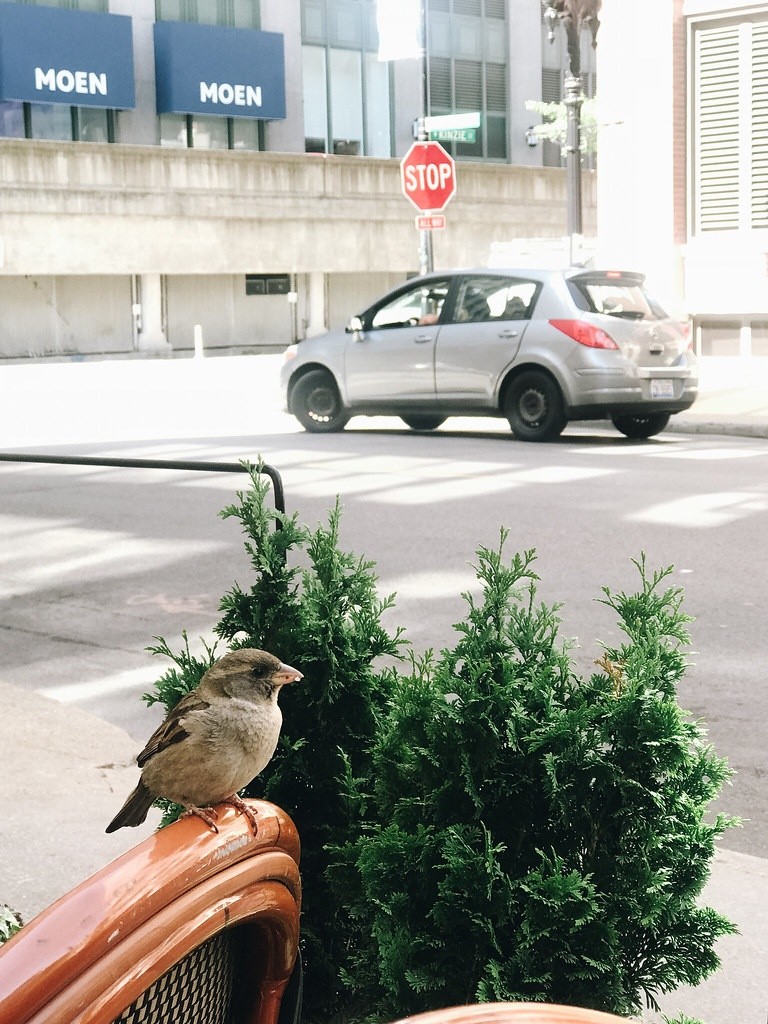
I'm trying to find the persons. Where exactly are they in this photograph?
[416,293,490,325]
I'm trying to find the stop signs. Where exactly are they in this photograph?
[400,139,457,212]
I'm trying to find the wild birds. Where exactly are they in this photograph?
[105,647,305,838]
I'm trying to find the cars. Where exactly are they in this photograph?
[278,266,699,444]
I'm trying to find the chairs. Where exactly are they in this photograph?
[0,799,303,1024]
[463,294,491,321]
